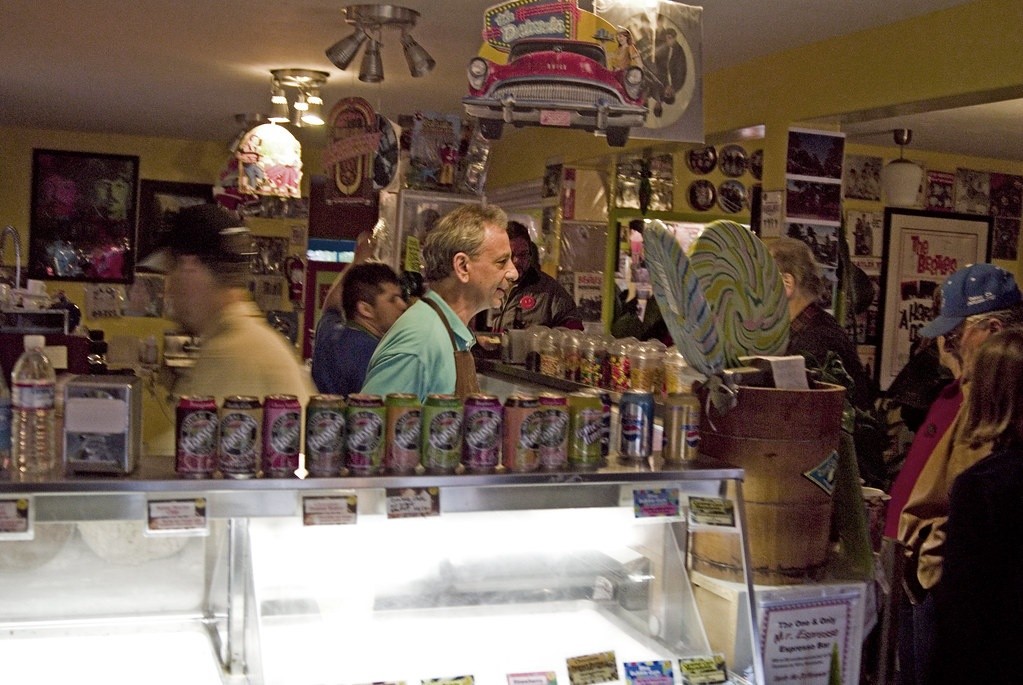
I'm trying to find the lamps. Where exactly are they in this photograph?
[267,61,327,128]
[882,127,924,208]
[325,5,434,82]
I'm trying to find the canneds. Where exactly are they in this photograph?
[616,388,655,462]
[174,389,612,480]
[661,391,700,467]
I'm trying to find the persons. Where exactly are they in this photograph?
[639,320,672,349]
[470,221,586,359]
[762,235,874,488]
[313,262,409,396]
[879,263,1023,685]
[359,203,518,406]
[150,202,309,457]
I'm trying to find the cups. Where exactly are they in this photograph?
[861,486,892,552]
[525,324,708,397]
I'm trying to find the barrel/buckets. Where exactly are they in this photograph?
[689,379,845,573]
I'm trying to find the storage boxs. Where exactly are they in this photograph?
[556,269,604,327]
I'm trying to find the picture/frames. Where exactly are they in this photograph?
[28,146,143,283]
[135,179,216,275]
[873,209,997,399]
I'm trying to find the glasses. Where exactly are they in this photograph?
[944,319,986,342]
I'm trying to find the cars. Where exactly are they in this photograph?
[462,36,650,148]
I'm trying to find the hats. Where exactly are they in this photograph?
[138,205,252,267]
[920,262,1021,338]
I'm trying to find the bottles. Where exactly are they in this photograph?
[10,334,56,474]
[0,368,10,470]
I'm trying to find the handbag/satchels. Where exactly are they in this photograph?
[840,237,874,311]
